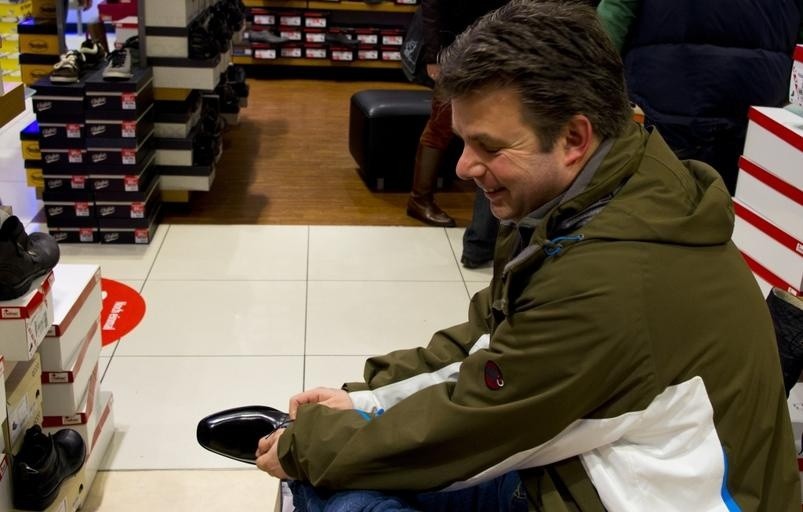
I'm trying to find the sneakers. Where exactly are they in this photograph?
[193,62,249,166]
[325,31,361,48]
[248,29,289,45]
[49,49,85,83]
[79,20,141,68]
[188,1,247,59]
[102,47,134,79]
[460,254,482,268]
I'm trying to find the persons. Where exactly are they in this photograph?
[197,0,803,512]
[401,0,795,269]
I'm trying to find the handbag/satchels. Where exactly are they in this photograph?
[400,7,443,90]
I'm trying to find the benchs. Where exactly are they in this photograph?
[347,88,476,194]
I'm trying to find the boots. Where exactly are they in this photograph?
[406,142,455,228]
[766,286,802,399]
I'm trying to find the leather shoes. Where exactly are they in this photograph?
[11,423,87,511]
[1,215,61,302]
[196,405,289,465]
